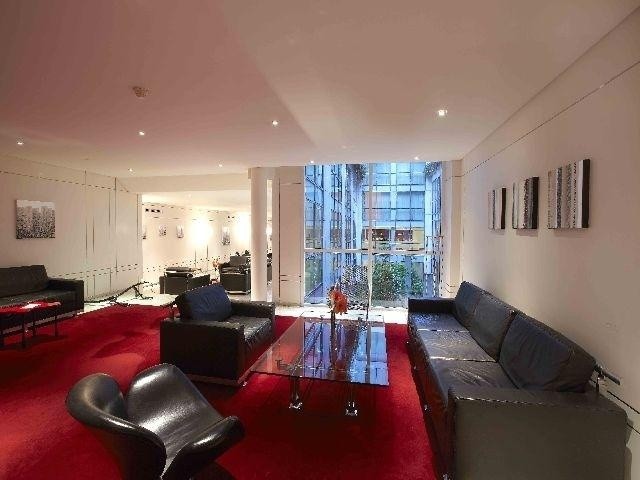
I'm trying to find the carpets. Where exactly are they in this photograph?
[2,303,437,480]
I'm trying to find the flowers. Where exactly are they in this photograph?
[328,287,347,314]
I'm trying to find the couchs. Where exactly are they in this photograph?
[406,281,627,480]
[0,264,85,333]
[219,260,271,293]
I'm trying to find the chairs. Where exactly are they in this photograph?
[67,363,246,479]
[160,267,210,295]
[219,256,249,290]
[159,285,275,394]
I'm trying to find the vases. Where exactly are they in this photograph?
[331,310,335,323]
